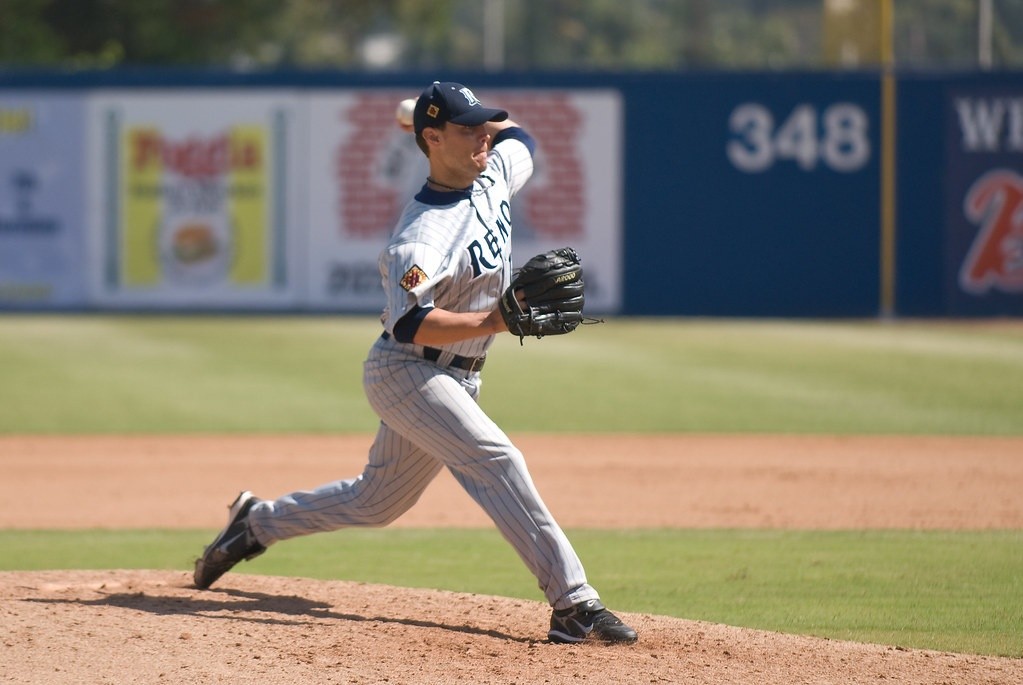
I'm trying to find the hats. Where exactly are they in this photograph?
[413,80,508,134]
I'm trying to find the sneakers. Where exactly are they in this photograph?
[548,599,638,646]
[194,491,267,589]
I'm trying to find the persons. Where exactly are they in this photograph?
[194,80,638,647]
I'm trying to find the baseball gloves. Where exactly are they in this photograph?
[497,246,585,346]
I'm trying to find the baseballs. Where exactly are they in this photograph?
[395,98,417,126]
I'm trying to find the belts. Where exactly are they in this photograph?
[381,330,485,372]
